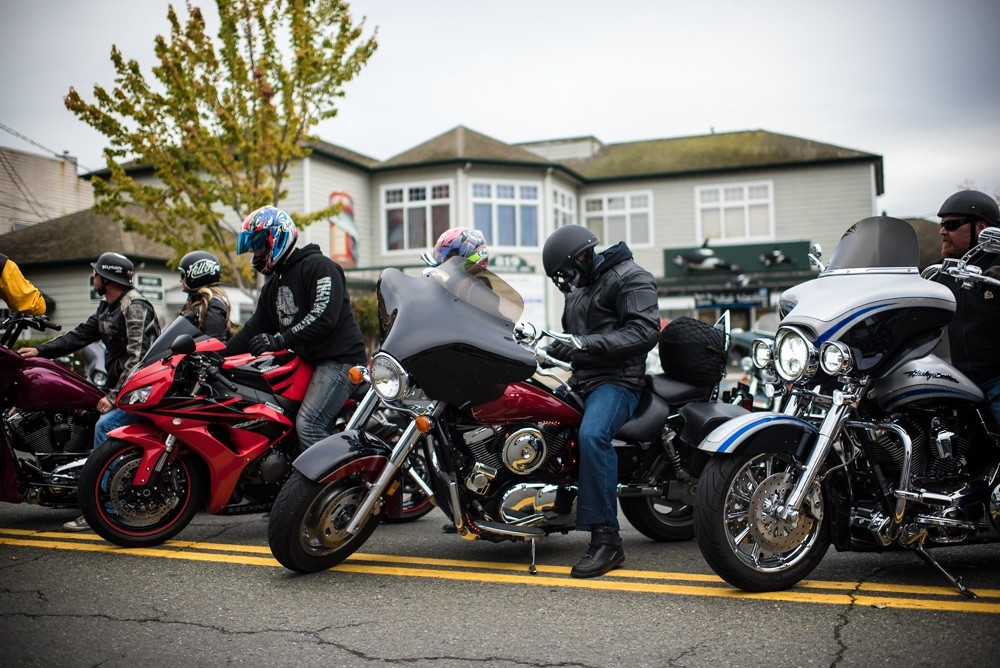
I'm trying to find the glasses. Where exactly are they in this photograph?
[178,271,186,280]
[937,218,970,232]
[92,268,98,277]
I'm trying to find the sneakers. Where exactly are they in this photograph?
[63,515,91,532]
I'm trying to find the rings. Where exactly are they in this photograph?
[102,408,104,411]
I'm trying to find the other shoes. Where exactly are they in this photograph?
[442,524,458,533]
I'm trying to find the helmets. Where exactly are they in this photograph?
[433,226,490,273]
[236,205,299,275]
[177,250,223,293]
[937,189,1000,227]
[542,223,600,293]
[90,251,136,288]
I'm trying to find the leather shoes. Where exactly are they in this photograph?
[571,533,626,578]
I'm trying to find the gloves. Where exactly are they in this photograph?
[545,333,589,362]
[246,332,285,356]
[41,293,55,317]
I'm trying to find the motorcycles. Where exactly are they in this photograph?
[1,217,1000,600]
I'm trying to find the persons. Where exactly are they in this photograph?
[179,250,240,342]
[432,227,492,534]
[0,253,46,316]
[217,204,367,449]
[16,253,160,530]
[542,225,661,576]
[926,189,1000,426]
